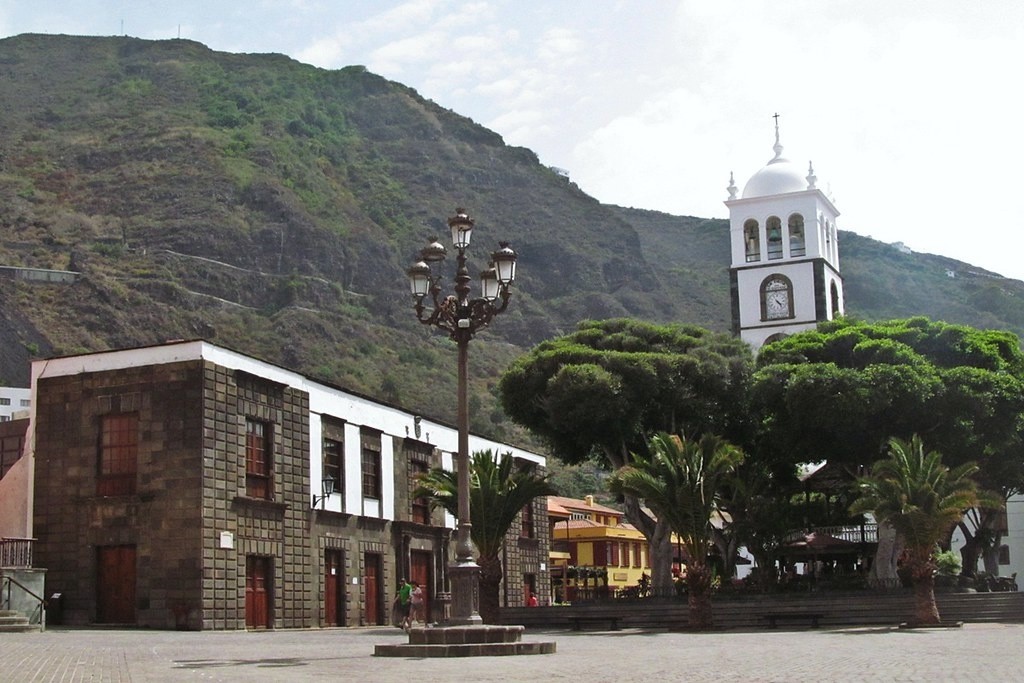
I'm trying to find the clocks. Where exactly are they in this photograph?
[760,273,796,322]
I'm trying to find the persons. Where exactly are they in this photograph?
[392,578,411,630]
[528,592,538,606]
[406,580,430,628]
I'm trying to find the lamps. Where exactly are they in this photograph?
[313,473,335,508]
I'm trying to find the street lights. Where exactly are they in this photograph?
[406,205,521,628]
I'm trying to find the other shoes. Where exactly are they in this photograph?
[397,624,403,629]
